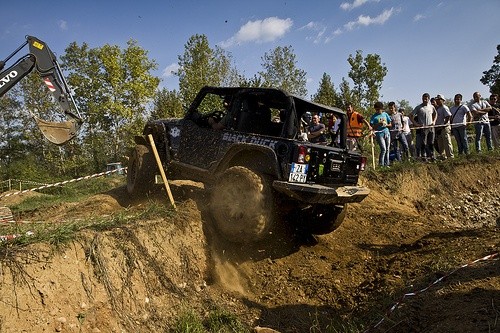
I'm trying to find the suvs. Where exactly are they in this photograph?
[125,85,371,244]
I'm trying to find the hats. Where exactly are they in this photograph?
[435,94,445,102]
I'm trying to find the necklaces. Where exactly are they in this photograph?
[478,101,483,110]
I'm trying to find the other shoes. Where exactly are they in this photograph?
[379,153,473,169]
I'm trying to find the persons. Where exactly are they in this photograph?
[370,102,392,169]
[271,110,341,146]
[488,94,500,150]
[469,91,494,154]
[208,95,242,129]
[450,94,473,158]
[410,93,454,164]
[388,101,416,164]
[345,102,372,152]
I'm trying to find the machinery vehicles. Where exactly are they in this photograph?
[0,34,85,145]
